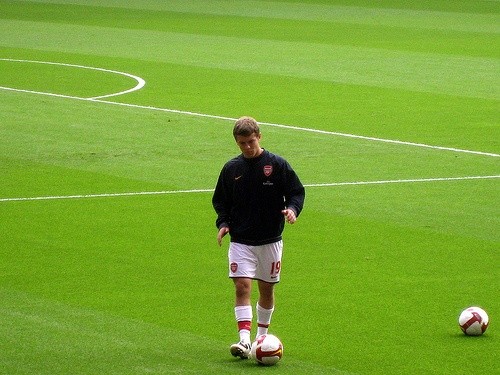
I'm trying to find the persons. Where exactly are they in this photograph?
[213,116,306,359]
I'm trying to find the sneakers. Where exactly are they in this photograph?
[229,341,251,358]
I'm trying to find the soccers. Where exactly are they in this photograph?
[458,307,489,336]
[251,334,283,365]
